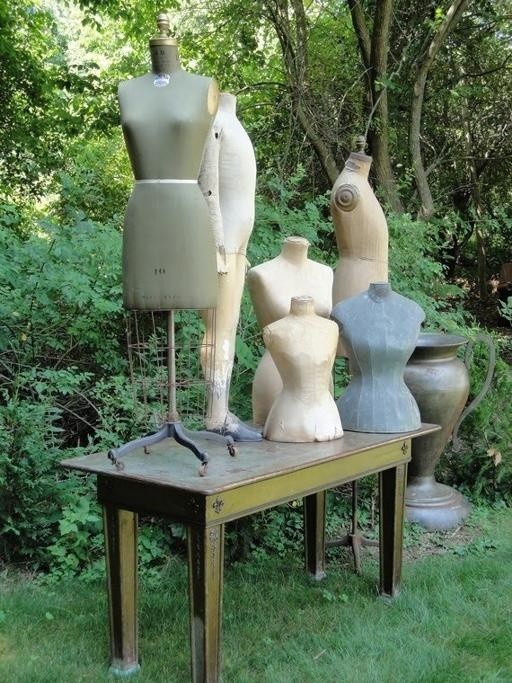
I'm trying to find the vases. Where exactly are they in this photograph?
[405,332,496,532]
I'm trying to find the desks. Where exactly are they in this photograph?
[61,419,442,683]
